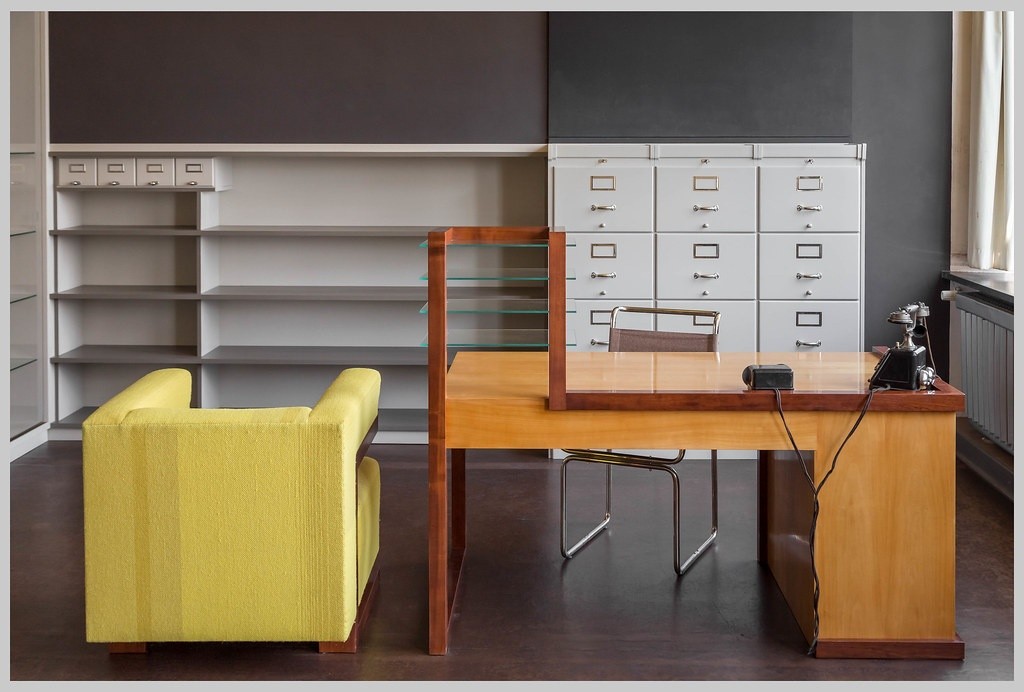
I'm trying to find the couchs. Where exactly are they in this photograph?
[82,368,382,655]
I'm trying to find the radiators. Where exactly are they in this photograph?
[955,291,1014,455]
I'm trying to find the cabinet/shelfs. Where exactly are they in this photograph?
[418,226,577,392]
[10,12,48,463]
[48,143,867,460]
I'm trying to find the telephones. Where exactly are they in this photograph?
[866,302,936,391]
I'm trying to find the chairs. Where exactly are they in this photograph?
[560,305,722,577]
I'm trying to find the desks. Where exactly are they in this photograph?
[419,346,967,661]
